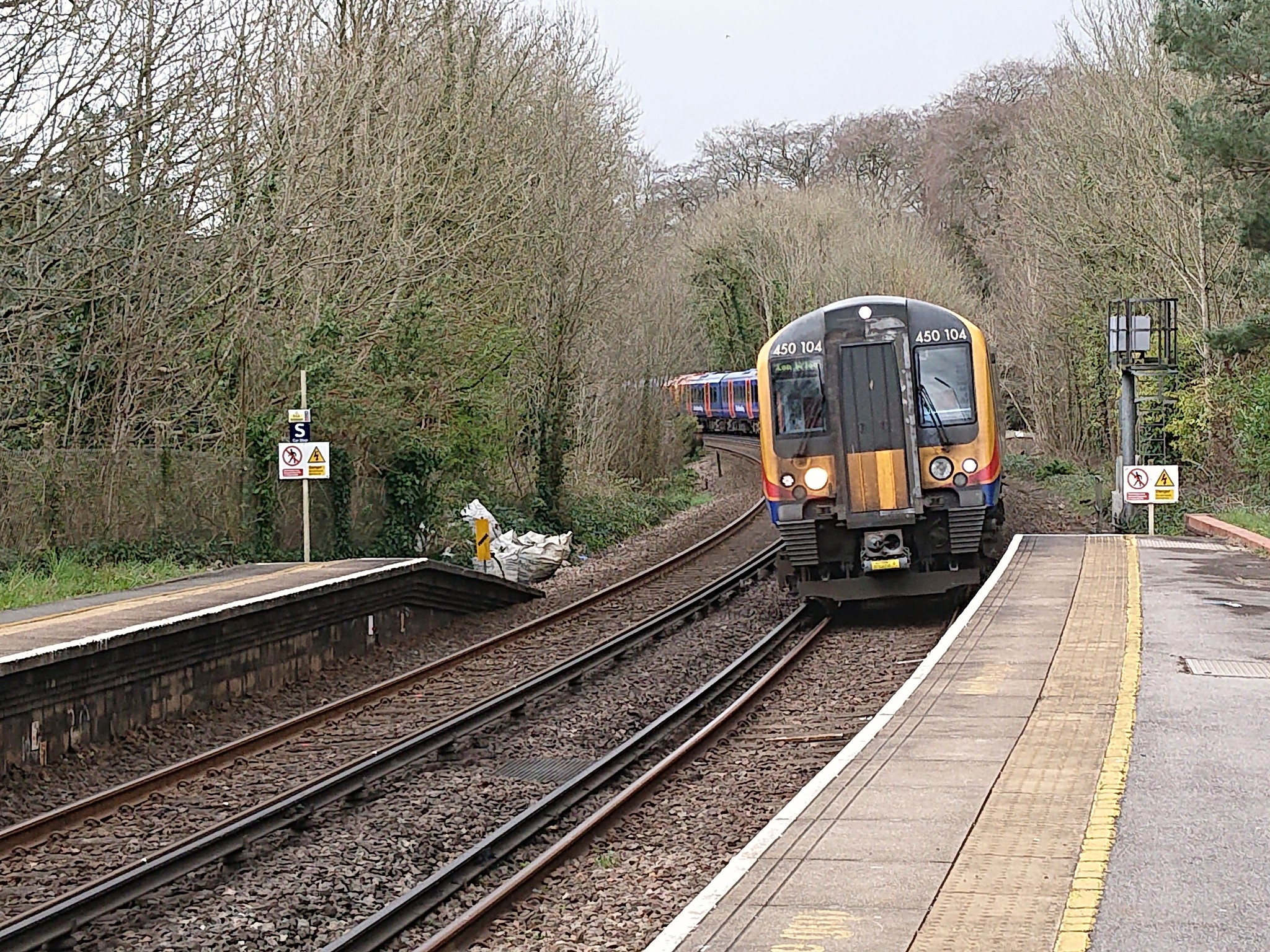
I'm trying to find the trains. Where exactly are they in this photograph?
[628,293,1007,618]
